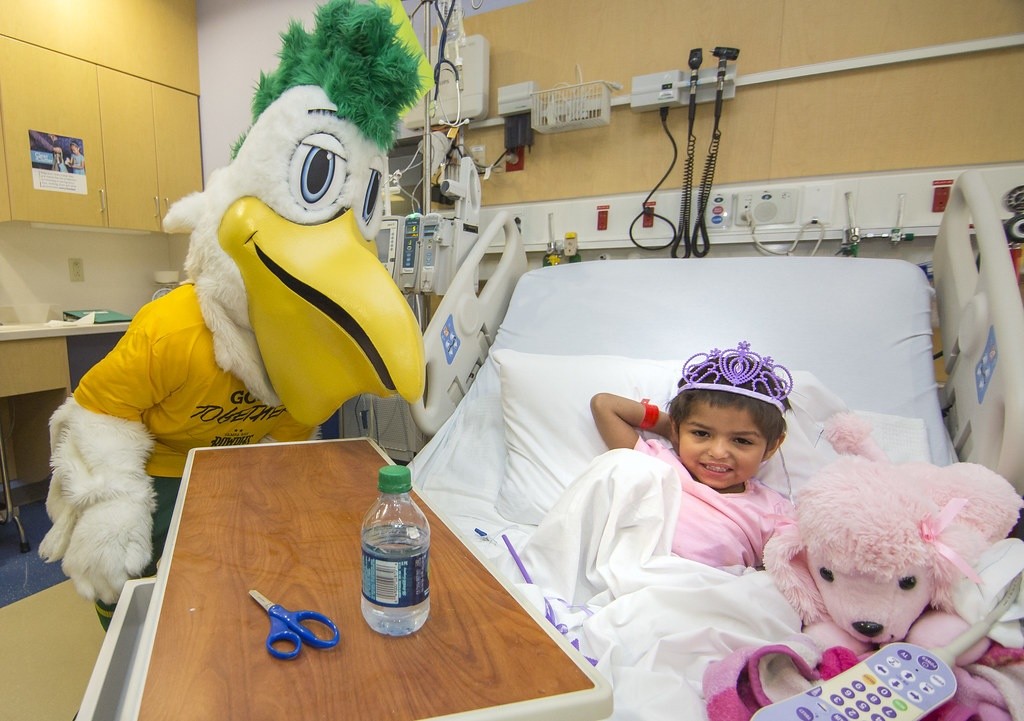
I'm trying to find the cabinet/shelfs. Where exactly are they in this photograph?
[97,63,203,234]
[0,33,109,227]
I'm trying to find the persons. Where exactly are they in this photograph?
[50,134,85,174]
[533,342,791,721]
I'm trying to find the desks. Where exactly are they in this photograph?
[75,437,616,721]
[0,322,131,554]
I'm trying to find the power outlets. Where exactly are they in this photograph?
[68,259,84,281]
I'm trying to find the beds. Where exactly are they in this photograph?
[405,169,1024,721]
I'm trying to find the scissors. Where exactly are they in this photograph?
[249,587,341,659]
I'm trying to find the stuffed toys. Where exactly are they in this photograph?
[38,1,433,629]
[764,412,1019,667]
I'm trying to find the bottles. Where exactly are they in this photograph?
[360,465,430,636]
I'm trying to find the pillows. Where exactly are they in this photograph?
[492,347,854,525]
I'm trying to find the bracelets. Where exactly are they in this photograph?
[641,399,659,429]
